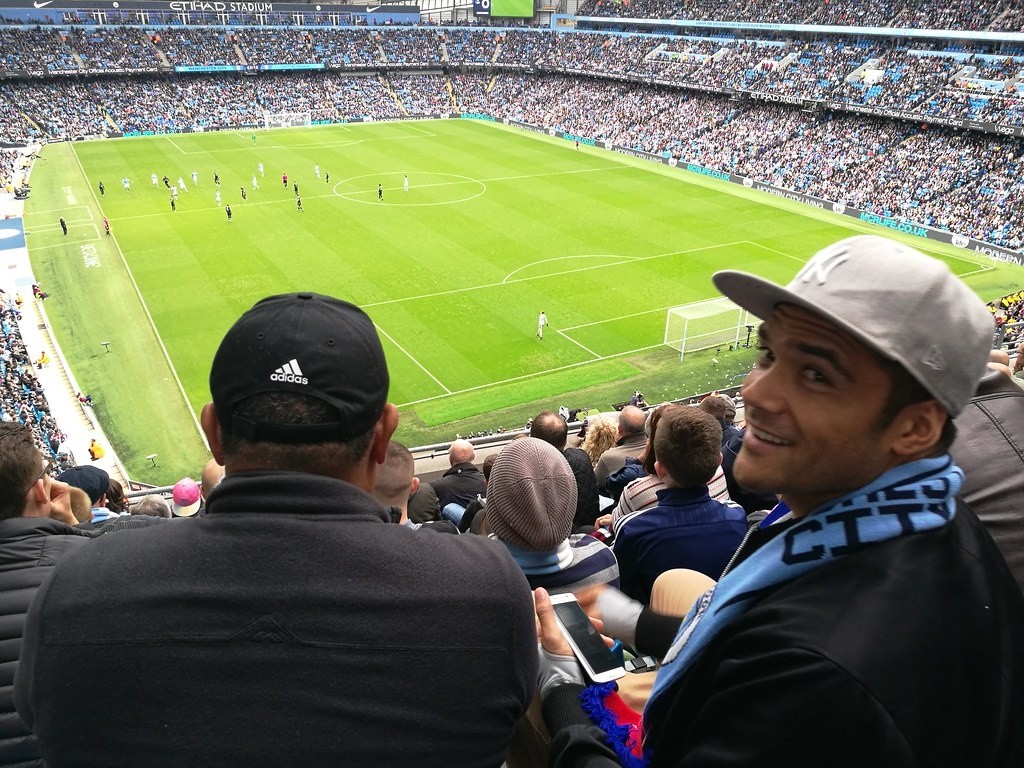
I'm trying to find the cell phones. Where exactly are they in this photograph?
[548,593,628,683]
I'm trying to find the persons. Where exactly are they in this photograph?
[483,437,620,598]
[579,394,782,533]
[612,406,750,606]
[462,0,1024,258]
[239,160,410,212]
[12,289,538,768]
[120,169,232,223]
[946,364,1024,585]
[531,234,1023,768]
[104,217,111,236]
[99,181,105,196]
[0,283,104,482]
[0,10,462,139]
[0,421,91,767]
[55,459,225,530]
[535,310,549,341]
[0,79,40,194]
[371,411,601,544]
[252,132,257,145]
[59,217,67,235]
[986,289,1023,382]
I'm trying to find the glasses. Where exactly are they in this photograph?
[25,457,53,495]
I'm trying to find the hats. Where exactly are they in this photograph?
[210,291,391,445]
[55,465,109,506]
[717,394,736,414]
[485,438,577,552]
[171,479,201,516]
[712,234,998,420]
